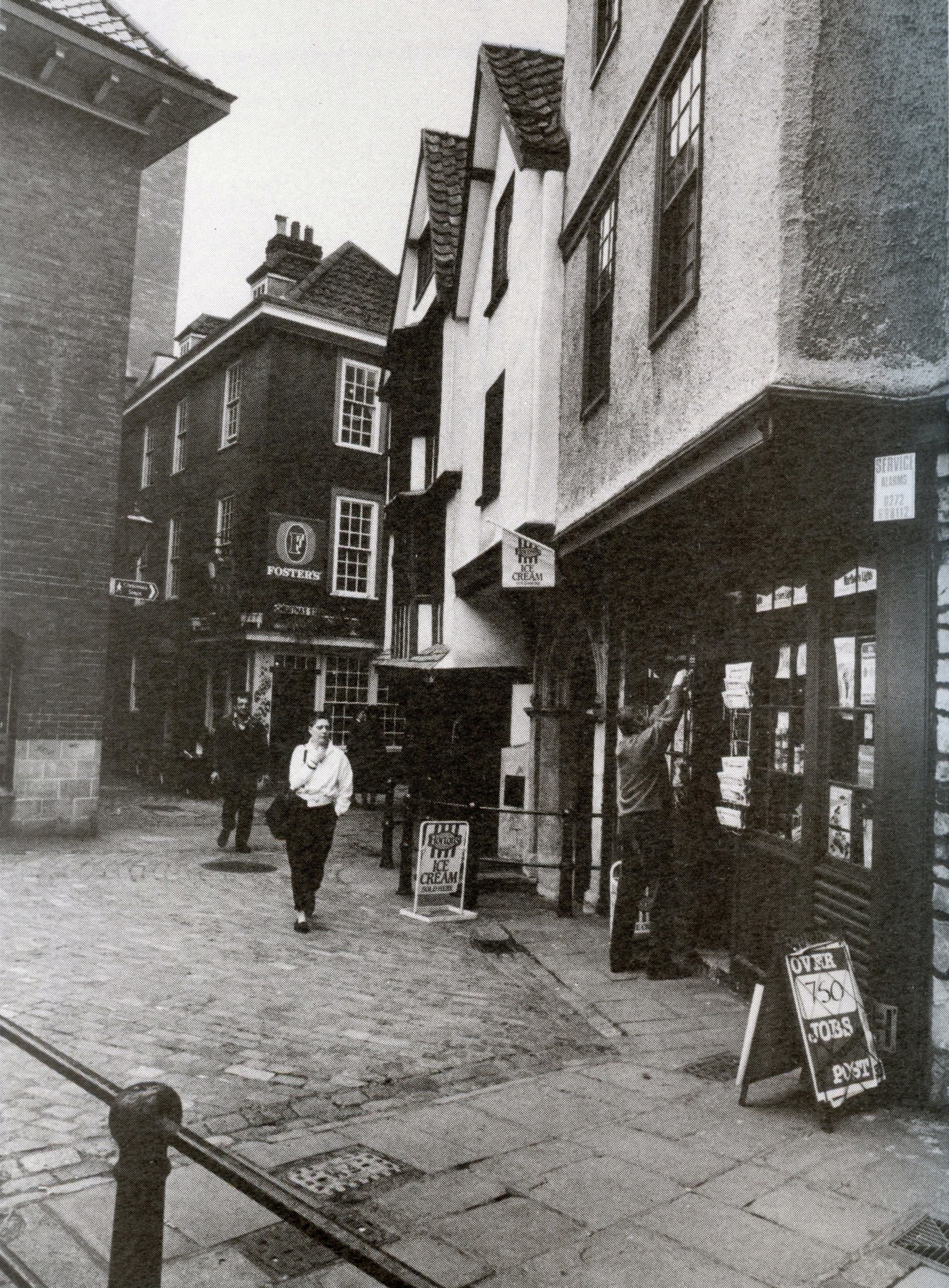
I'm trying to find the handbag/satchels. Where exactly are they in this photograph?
[265,791,308,842]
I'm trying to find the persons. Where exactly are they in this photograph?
[210,693,271,854]
[285,712,353,934]
[345,705,392,811]
[610,668,693,980]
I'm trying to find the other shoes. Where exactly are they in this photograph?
[234,844,252,853]
[303,893,314,917]
[609,949,648,972]
[293,918,309,933]
[645,954,694,980]
[216,824,235,847]
